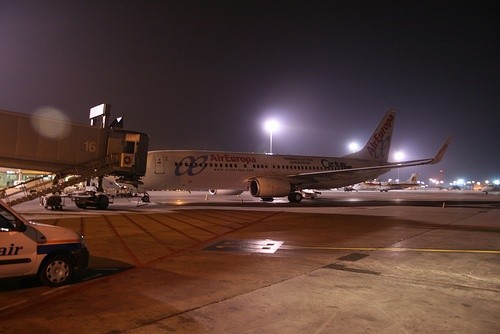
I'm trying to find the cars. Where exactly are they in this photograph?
[0,198,88,287]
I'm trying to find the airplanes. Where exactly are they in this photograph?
[352,172,423,193]
[90,105,451,202]
